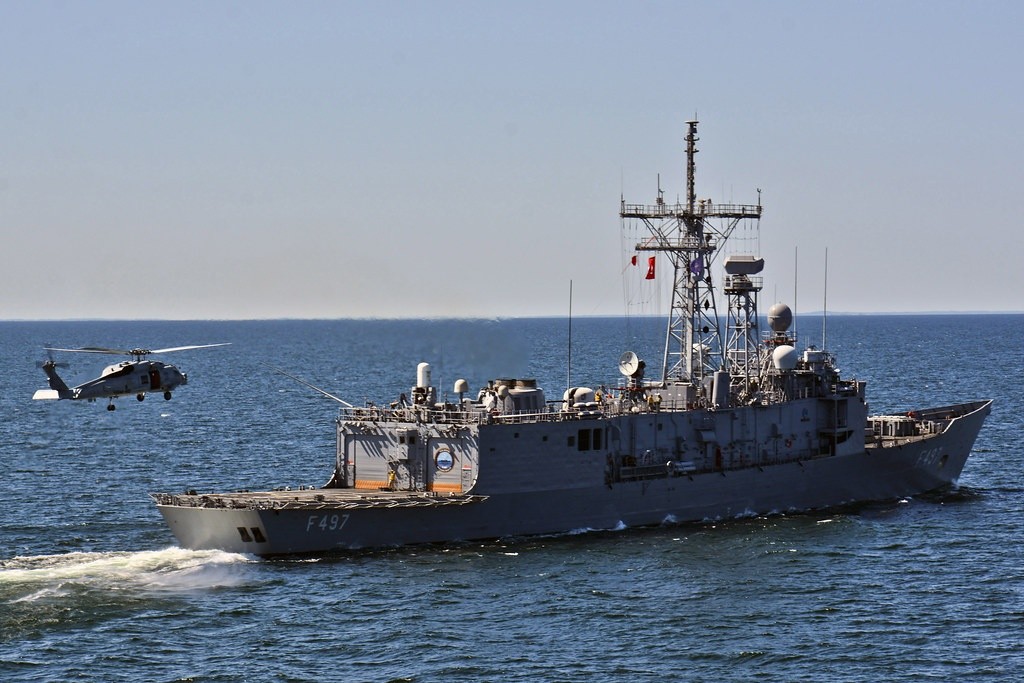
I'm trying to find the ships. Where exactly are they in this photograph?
[154,121,996,567]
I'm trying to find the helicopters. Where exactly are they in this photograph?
[28,342,234,413]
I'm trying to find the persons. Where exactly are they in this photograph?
[594,391,625,408]
[388,470,396,492]
[875,421,881,435]
[648,393,662,412]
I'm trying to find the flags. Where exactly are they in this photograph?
[645,257,655,279]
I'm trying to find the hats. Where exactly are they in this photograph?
[657,392,660,395]
